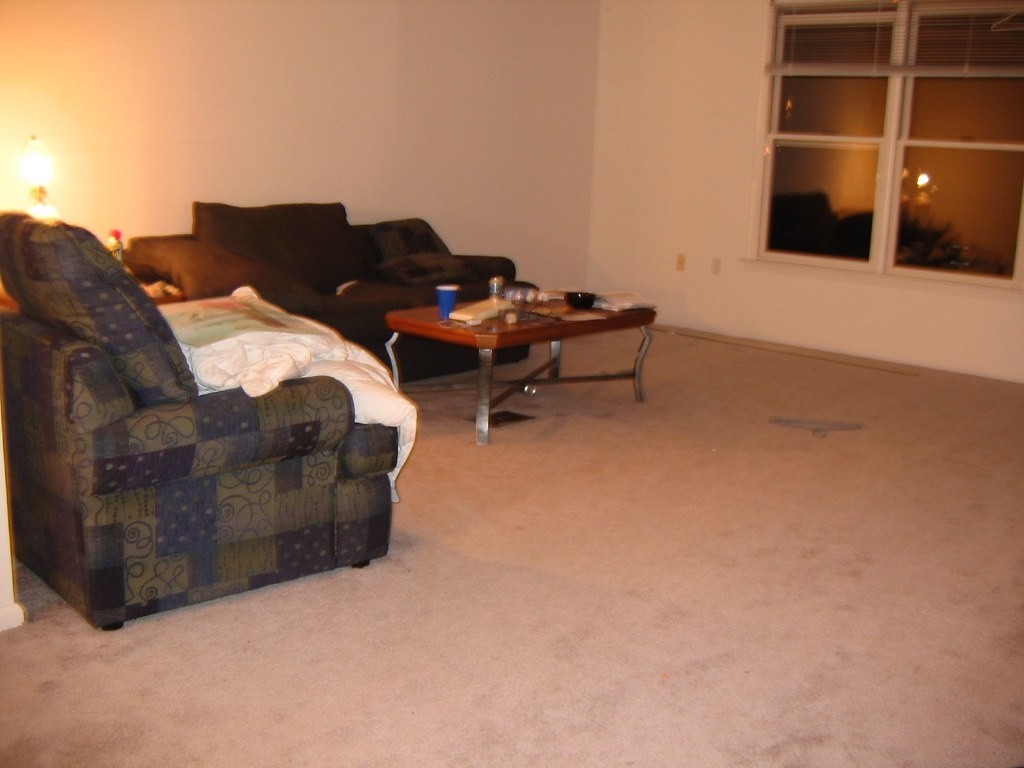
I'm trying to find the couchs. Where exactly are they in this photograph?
[123,233,539,383]
[0,310,420,631]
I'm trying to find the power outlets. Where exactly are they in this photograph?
[711,257,719,276]
[676,254,685,272]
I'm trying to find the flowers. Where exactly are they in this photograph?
[108,228,121,243]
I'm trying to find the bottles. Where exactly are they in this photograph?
[106,230,123,264]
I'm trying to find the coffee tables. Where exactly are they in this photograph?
[383,303,657,447]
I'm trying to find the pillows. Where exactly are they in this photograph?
[0,211,200,407]
[377,253,463,286]
[192,201,351,291]
[369,218,452,260]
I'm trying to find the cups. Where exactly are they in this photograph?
[435,286,458,319]
[489,276,503,299]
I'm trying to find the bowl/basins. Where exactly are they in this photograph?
[564,292,596,309]
